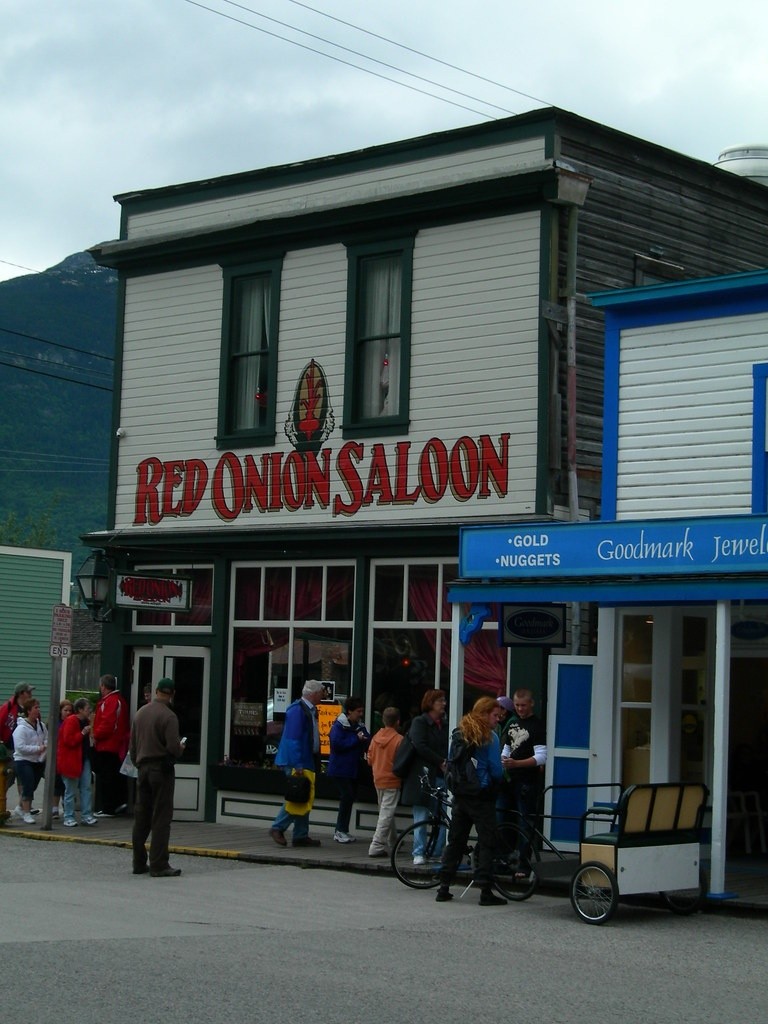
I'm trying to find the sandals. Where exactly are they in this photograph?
[514,864,531,878]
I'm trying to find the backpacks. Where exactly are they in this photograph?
[444,730,493,796]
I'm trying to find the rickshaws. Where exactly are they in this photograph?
[389,765,711,926]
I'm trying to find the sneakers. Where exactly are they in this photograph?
[1,802,127,825]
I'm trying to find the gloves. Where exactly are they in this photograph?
[81,726,91,735]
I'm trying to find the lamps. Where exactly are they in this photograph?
[76,549,115,623]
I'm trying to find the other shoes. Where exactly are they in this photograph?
[413,855,425,865]
[369,850,389,858]
[392,840,405,848]
[149,863,181,877]
[427,856,443,863]
[133,863,151,874]
[345,832,356,842]
[268,828,287,846]
[333,830,351,844]
[479,893,508,906]
[435,890,453,901]
[292,836,321,847]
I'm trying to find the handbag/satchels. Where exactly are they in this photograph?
[119,749,139,778]
[391,716,428,777]
[284,770,311,804]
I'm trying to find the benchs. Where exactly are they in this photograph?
[579,780,706,847]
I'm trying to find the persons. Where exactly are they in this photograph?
[327,697,368,843]
[366,707,405,858]
[11,698,48,824]
[144,683,151,702]
[42,700,73,819]
[129,678,185,877]
[58,697,97,826]
[0,681,40,816]
[409,689,448,865]
[268,680,321,847]
[92,675,128,818]
[436,685,547,906]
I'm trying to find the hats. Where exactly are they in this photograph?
[496,696,514,711]
[15,681,36,692]
[158,678,175,695]
